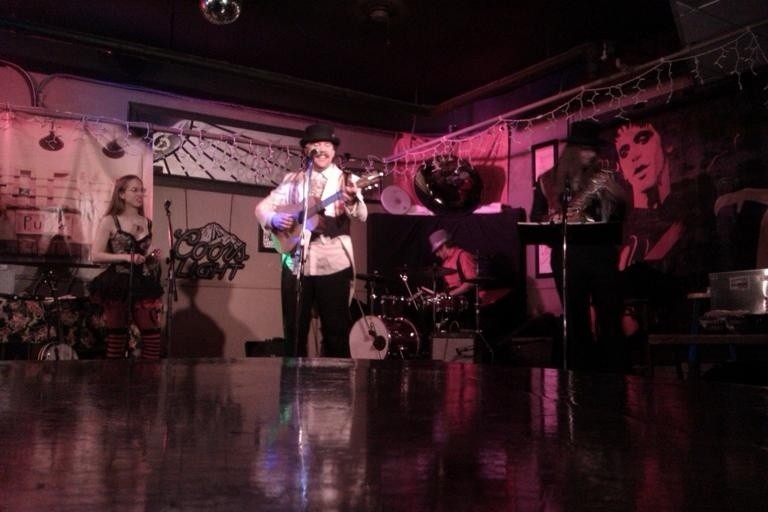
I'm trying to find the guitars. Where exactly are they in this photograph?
[270,168,386,254]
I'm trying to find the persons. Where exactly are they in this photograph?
[601,117,735,349]
[532,126,638,368]
[92,172,164,360]
[255,121,368,358]
[712,178,768,266]
[409,229,480,365]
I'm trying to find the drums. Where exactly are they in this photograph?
[348,316,421,361]
[436,297,469,312]
[382,295,419,321]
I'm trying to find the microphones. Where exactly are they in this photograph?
[561,177,571,200]
[307,148,321,165]
[164,199,171,213]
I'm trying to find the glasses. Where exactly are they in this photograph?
[126,187,146,192]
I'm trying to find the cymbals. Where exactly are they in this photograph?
[460,277,498,283]
[356,274,394,283]
[415,267,457,279]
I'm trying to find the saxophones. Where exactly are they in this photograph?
[555,156,620,224]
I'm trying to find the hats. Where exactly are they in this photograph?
[428,229,452,253]
[300,125,340,146]
